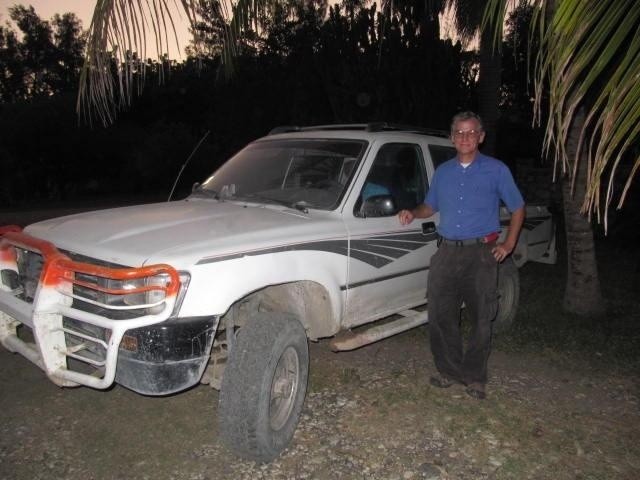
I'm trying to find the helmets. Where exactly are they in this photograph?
[453,130,479,139]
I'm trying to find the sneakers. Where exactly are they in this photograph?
[466,379,485,399]
[429,371,456,388]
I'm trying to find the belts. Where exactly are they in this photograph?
[443,233,499,247]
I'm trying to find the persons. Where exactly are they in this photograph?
[396,110,526,400]
[342,158,390,216]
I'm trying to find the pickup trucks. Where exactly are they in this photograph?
[0,120,558,461]
